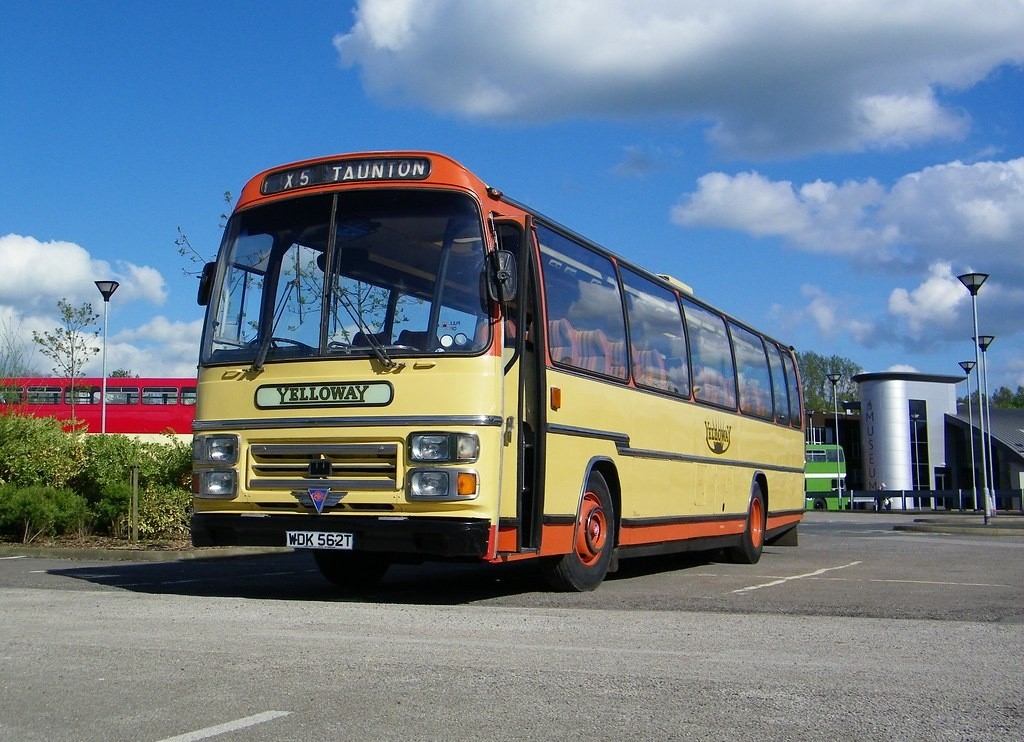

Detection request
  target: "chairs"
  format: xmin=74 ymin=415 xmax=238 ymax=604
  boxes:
xmin=395 ymin=330 xmax=439 ymax=352
xmin=475 ymin=317 xmax=774 ymax=421
xmin=350 ymin=332 xmax=390 ymax=356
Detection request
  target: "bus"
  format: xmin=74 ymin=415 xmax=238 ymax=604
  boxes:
xmin=1 ymin=377 xmax=197 ymax=446
xmin=804 ymin=443 xmax=848 ymax=511
xmin=188 ymin=150 xmax=806 ymax=591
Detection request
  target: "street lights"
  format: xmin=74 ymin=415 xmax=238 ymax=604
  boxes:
xmin=956 ymin=272 xmax=992 ymax=525
xmin=957 ymin=361 xmax=977 ymax=512
xmin=94 ymin=280 xmax=120 ymax=435
xmin=827 ymin=374 xmax=844 ymax=511
xmin=971 ymin=335 xmax=996 ymax=516
